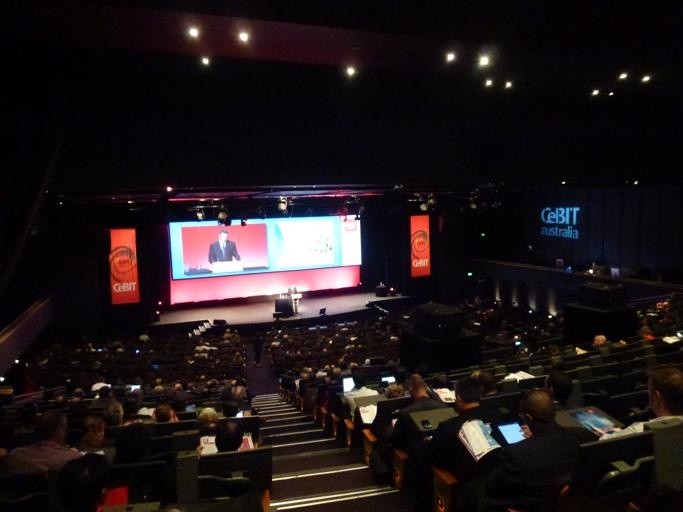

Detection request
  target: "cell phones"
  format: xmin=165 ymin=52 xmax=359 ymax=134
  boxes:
xmin=421 ymin=419 xmax=432 ymax=429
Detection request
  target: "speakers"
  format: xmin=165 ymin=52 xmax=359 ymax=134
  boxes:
xmin=213 ymin=319 xmax=226 ymax=324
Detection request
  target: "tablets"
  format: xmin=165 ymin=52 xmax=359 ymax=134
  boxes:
xmin=494 ymin=421 xmax=526 ymax=445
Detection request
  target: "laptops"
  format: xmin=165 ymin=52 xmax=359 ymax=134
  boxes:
xmin=342 ymin=376 xmax=355 ymax=396
xmin=131 ymin=384 xmax=141 ymax=392
xmin=235 ymin=411 xmax=244 ymax=417
xmin=381 ymin=376 xmax=395 ymax=384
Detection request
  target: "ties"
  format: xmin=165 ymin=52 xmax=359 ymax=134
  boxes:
xmin=223 ymin=244 xmax=225 ymax=256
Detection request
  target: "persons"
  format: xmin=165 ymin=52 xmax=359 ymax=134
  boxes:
xmin=2 ymin=321 xmax=269 ymax=512
xmin=270 ymin=294 xmax=682 ymax=512
xmin=208 ymin=230 xmax=241 ymax=264
